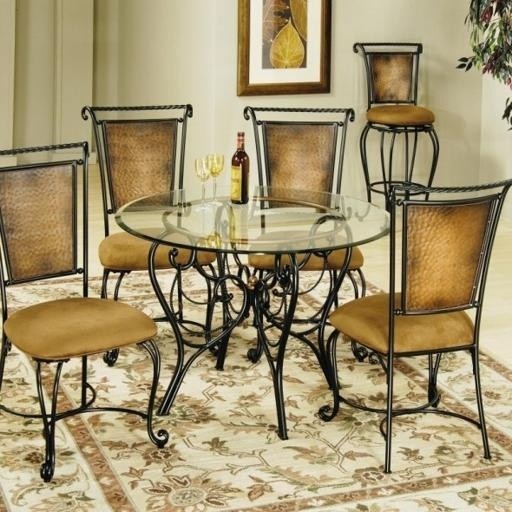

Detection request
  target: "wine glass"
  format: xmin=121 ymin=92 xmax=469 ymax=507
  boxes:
xmin=195 ymin=159 xmax=211 ymax=212
xmin=206 ymin=154 xmax=225 ymax=208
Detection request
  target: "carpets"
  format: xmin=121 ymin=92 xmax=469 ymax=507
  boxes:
xmin=1 ymin=261 xmax=510 ymax=512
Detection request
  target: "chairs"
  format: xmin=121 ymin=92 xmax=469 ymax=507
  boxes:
xmin=80 ymin=103 xmax=219 ymax=366
xmin=2 ymin=141 xmax=169 ymax=484
xmin=240 ymin=104 xmax=369 ymax=362
xmin=352 ymin=41 xmax=439 ymax=216
xmin=315 ymin=176 xmax=511 ymax=475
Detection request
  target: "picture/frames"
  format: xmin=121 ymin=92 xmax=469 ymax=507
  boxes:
xmin=236 ymin=0 xmax=333 ymax=100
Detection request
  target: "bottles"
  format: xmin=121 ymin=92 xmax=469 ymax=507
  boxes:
xmin=228 ymin=204 xmax=248 ymax=251
xmin=230 ymin=132 xmax=250 ymax=204
